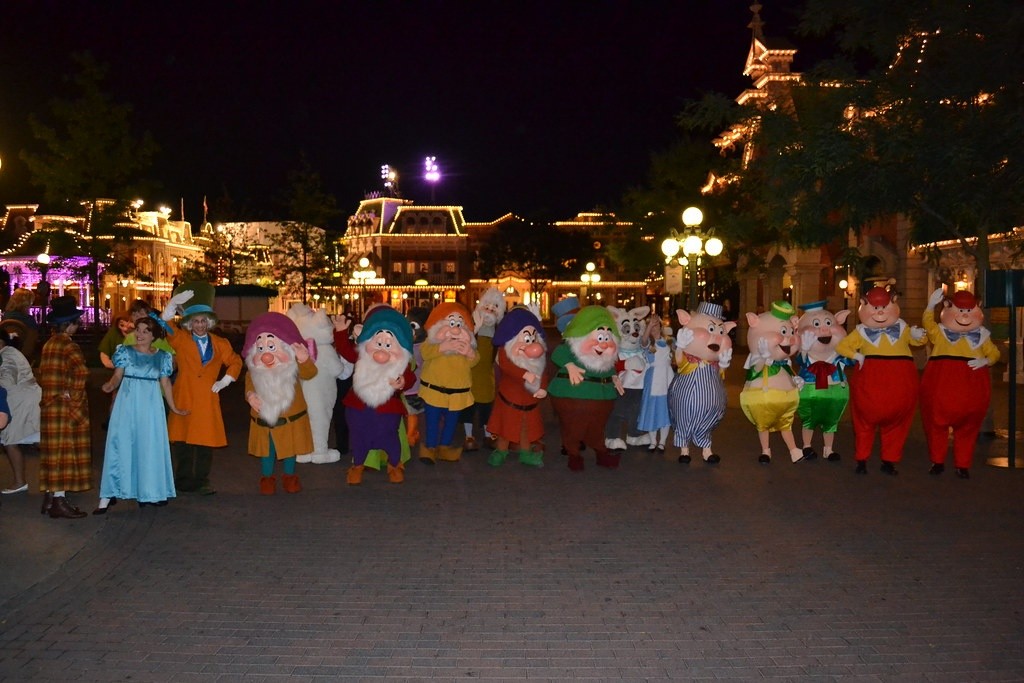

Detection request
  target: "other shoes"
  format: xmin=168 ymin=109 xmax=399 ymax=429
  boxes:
xmin=2 ymin=483 xmax=29 ymax=494
xmin=197 ymin=485 xmax=215 ymax=495
xmin=658 ymin=443 xmax=665 ymax=451
xmin=649 ymin=442 xmax=657 ymax=450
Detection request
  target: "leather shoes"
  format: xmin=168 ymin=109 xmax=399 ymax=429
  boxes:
xmin=41 ymin=492 xmax=55 ymax=514
xmin=51 ymin=496 xmax=88 ymax=518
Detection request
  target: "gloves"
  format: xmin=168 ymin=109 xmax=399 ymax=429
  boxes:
xmin=211 ymin=374 xmax=233 ymax=393
xmin=162 ymin=289 xmax=195 ymax=322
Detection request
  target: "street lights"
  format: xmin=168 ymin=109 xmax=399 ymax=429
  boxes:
xmin=579 ymin=262 xmax=602 ymax=308
xmin=350 ymin=258 xmax=376 ymax=326
xmin=38 ymin=251 xmax=50 ymax=337
xmin=661 ymin=206 xmax=723 ymax=313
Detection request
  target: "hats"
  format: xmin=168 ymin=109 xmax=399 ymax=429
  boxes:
xmin=174 ymin=281 xmax=219 ymax=328
xmin=44 ymin=296 xmax=85 ymax=324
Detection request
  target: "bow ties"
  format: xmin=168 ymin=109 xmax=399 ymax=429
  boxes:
xmin=193 ymin=334 xmax=206 ymax=344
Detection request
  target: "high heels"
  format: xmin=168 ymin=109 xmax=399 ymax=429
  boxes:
xmin=92 ymin=496 xmax=117 ymax=515
xmin=138 ymin=500 xmax=168 ymax=509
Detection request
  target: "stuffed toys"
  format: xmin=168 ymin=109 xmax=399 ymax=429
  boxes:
xmin=738 ymin=299 xmax=808 ymax=465
xmin=793 ymin=298 xmax=850 ymax=462
xmin=404 ymin=306 xmax=428 ymax=448
xmin=665 ymin=302 xmax=736 ymax=463
xmin=918 ymin=286 xmax=1000 ymax=480
xmin=835 ymin=277 xmax=930 ymax=476
xmin=287 ymin=301 xmax=355 ymax=464
xmin=604 ymin=304 xmax=658 ymax=453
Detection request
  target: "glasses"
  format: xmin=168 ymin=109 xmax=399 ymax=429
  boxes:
xmin=192 ymin=318 xmax=208 ymax=325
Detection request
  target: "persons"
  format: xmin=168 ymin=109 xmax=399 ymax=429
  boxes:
xmin=36 ymin=294 xmax=93 ymax=518
xmin=462 ymin=287 xmax=506 ymax=450
xmin=330 ymin=302 xmax=417 ymax=484
xmin=37 ymin=312 xmax=40 ymax=323
xmin=418 ymin=301 xmax=480 ymax=464
xmin=241 ymin=311 xmax=317 ymax=495
xmin=97 ymin=300 xmax=163 ymax=411
xmin=91 ymin=320 xmax=192 ymax=515
xmin=487 ymin=307 xmax=555 ymax=468
xmin=0 ymin=288 xmax=42 ymax=496
xmin=639 ymin=315 xmax=676 ymax=456
xmin=159 ymin=290 xmax=243 ymax=492
xmin=547 ymin=295 xmax=626 ymax=474
